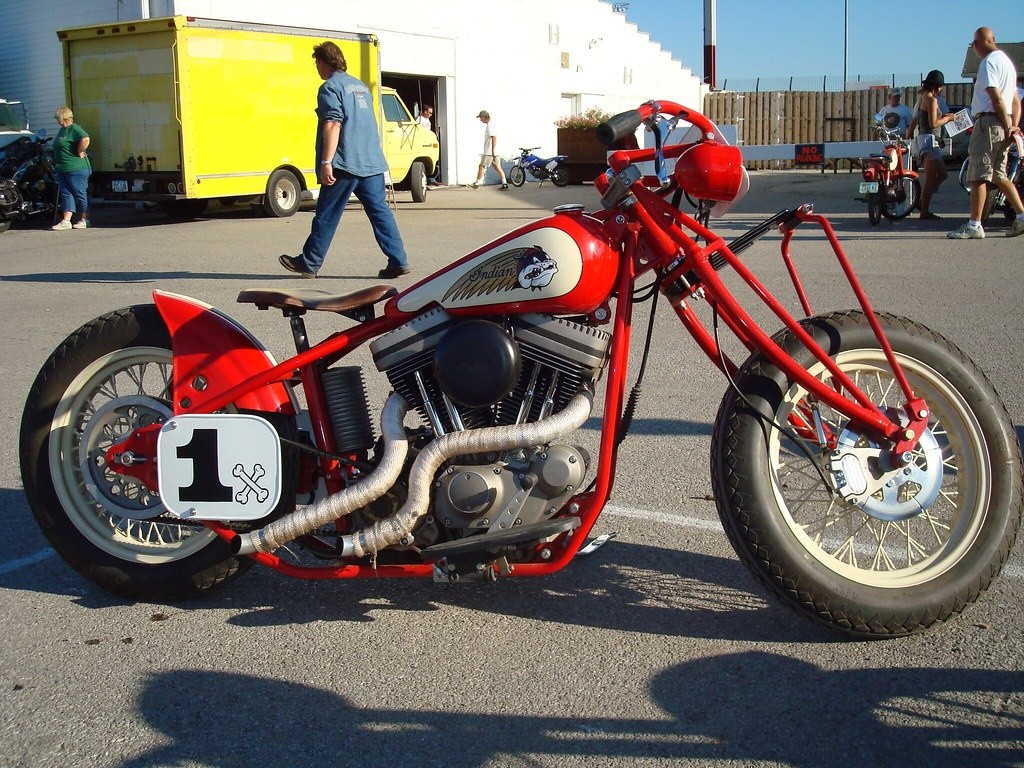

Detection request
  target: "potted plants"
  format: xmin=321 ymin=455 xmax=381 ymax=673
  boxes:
xmin=558 ymin=128 xmax=638 ymax=180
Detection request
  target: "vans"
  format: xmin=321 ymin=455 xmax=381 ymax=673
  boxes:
xmin=0 ymin=98 xmax=38 ymax=158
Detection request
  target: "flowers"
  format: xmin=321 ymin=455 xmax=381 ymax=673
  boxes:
xmin=554 ymin=105 xmax=613 ymax=129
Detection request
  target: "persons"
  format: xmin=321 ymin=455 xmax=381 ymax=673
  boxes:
xmin=909 ymin=69 xmax=958 ymax=220
xmin=415 ymin=105 xmax=439 ymax=186
xmin=51 ymin=107 xmax=92 ymax=230
xmin=466 ymin=110 xmax=508 ymax=191
xmin=278 ymin=42 xmax=409 ymax=278
xmin=874 ymin=87 xmax=911 ymax=139
xmin=949 ymin=27 xmax=1024 ymax=240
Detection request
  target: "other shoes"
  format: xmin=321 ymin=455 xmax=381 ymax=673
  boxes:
xmin=498 ymin=184 xmax=508 ymax=190
xmin=920 ymin=212 xmax=940 ymax=219
xmin=912 ymin=197 xmax=922 ymax=211
xmin=466 ymin=182 xmax=479 ymax=190
xmin=431 ymin=180 xmax=442 ymax=186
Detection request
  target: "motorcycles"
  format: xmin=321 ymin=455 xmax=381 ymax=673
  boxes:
xmin=15 ymin=99 xmax=1024 ymax=642
xmin=509 ymin=146 xmax=572 ymax=189
xmin=959 ymin=129 xmax=1024 ymax=228
xmin=852 ymin=113 xmax=923 ymax=228
xmin=0 ymin=127 xmax=63 ymax=232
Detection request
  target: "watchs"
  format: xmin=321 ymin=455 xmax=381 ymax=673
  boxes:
xmin=321 ymin=160 xmax=331 ymax=165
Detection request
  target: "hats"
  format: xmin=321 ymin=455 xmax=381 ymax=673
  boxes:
xmin=926 ymin=70 xmax=944 ymax=84
xmin=476 ymin=110 xmax=490 ymax=117
xmin=887 ymin=87 xmax=902 ymax=95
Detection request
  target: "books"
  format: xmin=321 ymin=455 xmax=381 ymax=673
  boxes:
xmin=1012 ymin=134 xmax=1024 ymax=157
xmin=944 ymin=109 xmax=973 ymax=137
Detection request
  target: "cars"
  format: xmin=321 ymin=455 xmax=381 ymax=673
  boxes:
xmin=939 ymin=106 xmax=975 ymax=170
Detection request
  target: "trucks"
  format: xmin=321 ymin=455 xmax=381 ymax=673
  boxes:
xmin=55 ymin=13 xmax=441 ymax=218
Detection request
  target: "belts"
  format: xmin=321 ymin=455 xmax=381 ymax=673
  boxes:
xmin=975 ymin=112 xmax=997 ymax=120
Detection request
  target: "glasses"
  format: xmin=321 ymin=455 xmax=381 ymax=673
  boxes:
xmin=426 ymin=111 xmax=433 ymax=114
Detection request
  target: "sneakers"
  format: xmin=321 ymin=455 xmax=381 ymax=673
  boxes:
xmin=278 ymin=254 xmax=315 ymax=278
xmin=73 ymin=220 xmax=86 ymax=229
xmin=1006 ymin=219 xmax=1024 ymax=236
xmin=378 ymin=265 xmax=410 ymax=277
xmin=947 ymin=223 xmax=985 ymax=239
xmin=52 ymin=220 xmax=72 ymax=230
